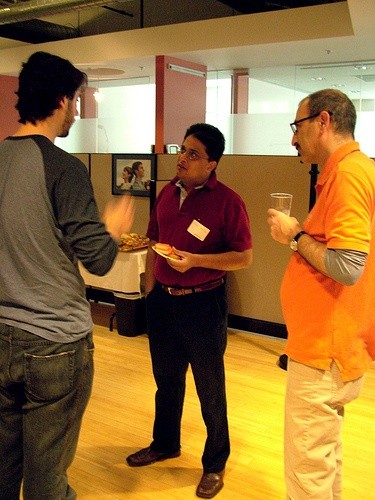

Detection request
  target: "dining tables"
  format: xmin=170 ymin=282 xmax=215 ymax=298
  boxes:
xmin=77 ymin=249 xmax=148 ymax=336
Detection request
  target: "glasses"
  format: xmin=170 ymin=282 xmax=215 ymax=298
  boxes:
xmin=290 ymin=111 xmax=333 ymax=135
xmin=177 ymin=146 xmax=208 ymax=161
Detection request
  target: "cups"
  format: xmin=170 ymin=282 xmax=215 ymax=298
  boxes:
xmin=270 ymin=193 xmax=293 ymax=216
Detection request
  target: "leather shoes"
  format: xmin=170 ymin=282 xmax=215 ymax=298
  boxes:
xmin=196 ymin=465 xmax=225 ymax=499
xmin=126 ymin=445 xmax=180 ymax=467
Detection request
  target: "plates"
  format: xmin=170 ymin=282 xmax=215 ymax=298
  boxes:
xmin=117 ymin=245 xmax=149 ymax=251
xmin=152 ymin=245 xmax=180 ymax=261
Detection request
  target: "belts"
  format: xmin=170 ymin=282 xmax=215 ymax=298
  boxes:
xmin=159 ymin=278 xmax=224 ymax=296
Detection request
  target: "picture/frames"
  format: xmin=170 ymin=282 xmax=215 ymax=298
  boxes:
xmin=111 ymin=153 xmax=157 ymax=196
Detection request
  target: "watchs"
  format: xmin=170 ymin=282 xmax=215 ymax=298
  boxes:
xmin=288 ymin=230 xmax=306 ymax=252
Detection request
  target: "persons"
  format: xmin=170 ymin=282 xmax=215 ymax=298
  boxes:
xmin=0 ymin=50 xmax=116 ymax=499
xmin=266 ymin=88 xmax=373 ymax=500
xmin=125 ymin=124 xmax=253 ymax=498
xmin=118 ymin=167 xmax=134 ymax=190
xmin=130 ymin=161 xmax=146 ymax=190
